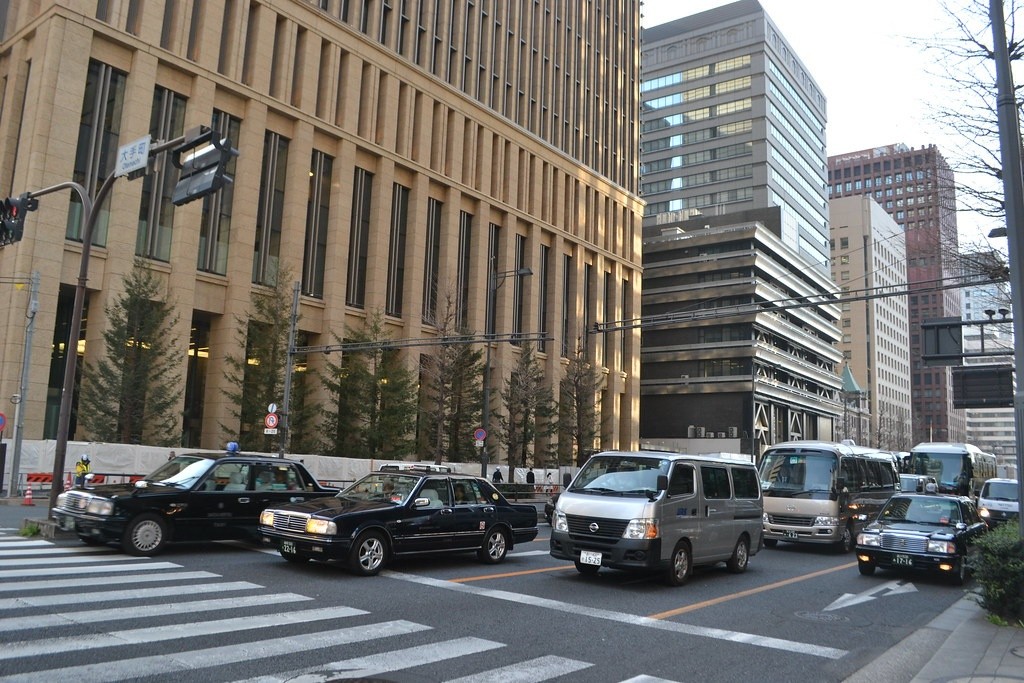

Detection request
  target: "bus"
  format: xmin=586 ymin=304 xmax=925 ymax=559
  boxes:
xmin=755 ymin=439 xmax=998 ymax=552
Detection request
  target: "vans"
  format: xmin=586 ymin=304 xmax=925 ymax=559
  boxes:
xmin=974 ymin=477 xmax=1019 ymax=531
xmin=549 ymin=450 xmax=764 ymax=587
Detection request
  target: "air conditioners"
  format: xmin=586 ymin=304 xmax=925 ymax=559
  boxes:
xmin=728 ymin=426 xmax=737 ymax=437
xmin=706 ymin=432 xmax=714 ymax=438
xmin=681 ymin=375 xmax=689 ymax=378
xmin=718 ymin=432 xmax=726 ymax=438
xmin=699 ymin=253 xmax=707 ymax=257
xmin=696 ymin=427 xmax=706 ymax=438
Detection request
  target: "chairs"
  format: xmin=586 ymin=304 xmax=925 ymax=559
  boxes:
xmin=205 ymin=470 xmax=286 ymax=491
xmin=467 ymin=492 xmax=477 ymax=503
xmin=420 ymin=489 xmax=444 ymax=507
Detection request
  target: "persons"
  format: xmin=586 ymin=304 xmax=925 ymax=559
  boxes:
xmin=455 ymin=484 xmax=467 ymax=505
xmin=493 ymin=467 xmax=503 ymax=483
xmin=76 ymin=455 xmax=90 ymax=485
xmin=256 ymin=469 xmax=296 ymax=490
xmin=378 ymin=480 xmax=396 ymax=498
xmin=526 ymin=467 xmax=535 ymax=483
xmin=168 ymin=451 xmax=180 ymax=476
xmin=546 ymin=473 xmax=553 ymax=496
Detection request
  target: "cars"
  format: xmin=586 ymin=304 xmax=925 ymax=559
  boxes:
xmin=855 ymin=491 xmax=990 ymax=587
xmin=256 ymin=463 xmax=539 ymax=577
xmin=51 ymin=441 xmax=345 ymax=557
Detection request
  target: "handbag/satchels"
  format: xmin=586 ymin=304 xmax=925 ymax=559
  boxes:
xmin=549 ymin=475 xmax=554 ymax=484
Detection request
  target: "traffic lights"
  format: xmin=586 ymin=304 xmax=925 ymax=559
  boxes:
xmin=170 ymin=138 xmax=240 ymax=206
xmin=0 ymin=197 xmax=26 ymax=247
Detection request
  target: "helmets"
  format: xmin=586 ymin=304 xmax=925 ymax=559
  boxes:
xmin=80 ymin=453 xmax=92 ymax=462
xmin=496 ymin=466 xmax=501 ymax=471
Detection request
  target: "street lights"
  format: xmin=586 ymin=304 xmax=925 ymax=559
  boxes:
xmin=844 ymin=395 xmax=867 ymax=438
xmin=481 ymin=267 xmax=533 ymax=478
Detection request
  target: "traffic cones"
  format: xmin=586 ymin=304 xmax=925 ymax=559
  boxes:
xmin=21 ymin=480 xmax=35 ymax=505
xmin=64 ymin=472 xmax=71 ymax=491
